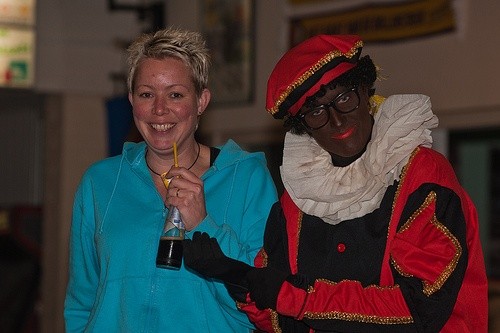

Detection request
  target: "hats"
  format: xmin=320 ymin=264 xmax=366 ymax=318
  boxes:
xmin=264 ymin=34 xmax=366 ymax=121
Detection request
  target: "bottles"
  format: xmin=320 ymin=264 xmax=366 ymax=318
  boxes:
xmin=155 ymin=205 xmax=186 ymax=271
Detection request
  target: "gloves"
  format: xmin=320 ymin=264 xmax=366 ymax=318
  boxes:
xmin=242 ymin=266 xmax=284 ymax=310
xmin=181 ymin=231 xmax=234 ymax=285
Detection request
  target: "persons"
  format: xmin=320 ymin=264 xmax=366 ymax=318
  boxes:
xmin=183 ymin=35 xmax=488 ymax=333
xmin=64 ymin=27 xmax=277 ymax=333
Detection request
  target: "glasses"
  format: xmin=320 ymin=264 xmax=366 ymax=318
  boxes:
xmin=296 ymin=81 xmax=363 ymax=131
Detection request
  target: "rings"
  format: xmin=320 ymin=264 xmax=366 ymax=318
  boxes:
xmin=175 ymin=188 xmax=181 ymax=198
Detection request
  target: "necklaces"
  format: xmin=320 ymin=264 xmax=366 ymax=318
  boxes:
xmin=144 ymin=141 xmax=201 ymax=177
